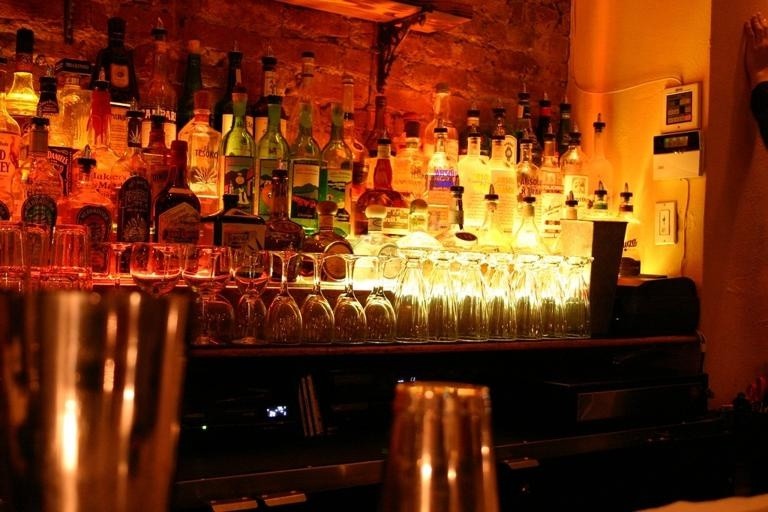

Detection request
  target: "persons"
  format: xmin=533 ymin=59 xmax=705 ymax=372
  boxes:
xmin=743 ymin=11 xmax=768 ymax=146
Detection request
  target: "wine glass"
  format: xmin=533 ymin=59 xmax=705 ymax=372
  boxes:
xmin=1 ymin=218 xmax=588 ymax=347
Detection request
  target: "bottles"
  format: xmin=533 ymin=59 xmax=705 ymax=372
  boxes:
xmin=1 ymin=16 xmax=643 ymax=288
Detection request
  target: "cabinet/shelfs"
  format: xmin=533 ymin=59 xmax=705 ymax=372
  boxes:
xmin=167 ymin=283 xmax=716 ymax=512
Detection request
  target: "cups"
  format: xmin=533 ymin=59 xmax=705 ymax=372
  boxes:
xmin=2 ymin=288 xmax=186 ymax=512
xmin=380 ymin=378 xmax=501 ymax=512
